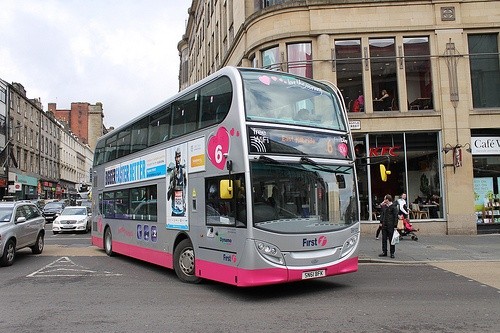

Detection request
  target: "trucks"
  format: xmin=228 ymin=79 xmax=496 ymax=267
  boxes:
xmin=74 ymin=183 xmax=92 ymax=207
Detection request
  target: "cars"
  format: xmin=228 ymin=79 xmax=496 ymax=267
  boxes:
xmin=51 ymin=205 xmax=92 ymax=235
xmin=30 ymin=198 xmax=71 ymax=212
xmin=41 ymin=201 xmax=65 ymax=224
xmin=81 ymin=199 xmax=92 ymax=213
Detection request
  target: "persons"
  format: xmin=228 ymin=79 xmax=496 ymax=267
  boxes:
xmin=414 ymin=194 xmax=439 ymax=218
xmin=297 ymin=109 xmax=310 ymax=120
xmin=368 ymin=195 xmax=380 ymax=220
xmin=376 ymin=201 xmax=388 ymax=240
xmin=378 ymin=90 xmax=392 ymax=112
xmin=378 ymin=195 xmax=398 ymax=258
xmin=396 ymin=193 xmax=409 ymax=240
xmin=270 ymin=187 xmax=281 ymax=207
xmin=167 ymin=147 xmax=186 ymax=216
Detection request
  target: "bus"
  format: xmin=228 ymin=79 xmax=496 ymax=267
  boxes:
xmin=89 ymin=64 xmax=393 ymax=291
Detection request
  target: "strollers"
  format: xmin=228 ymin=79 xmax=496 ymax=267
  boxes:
xmin=396 ymin=213 xmax=420 ymax=241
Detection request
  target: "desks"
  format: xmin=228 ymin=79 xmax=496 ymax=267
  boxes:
xmin=373 ymin=99 xmax=383 ymax=111
xmin=410 ymin=98 xmax=431 ymax=109
xmin=419 ymin=204 xmax=437 ymax=219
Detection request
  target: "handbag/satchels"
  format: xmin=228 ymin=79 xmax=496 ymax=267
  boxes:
xmin=391 ymin=229 xmax=400 ymax=245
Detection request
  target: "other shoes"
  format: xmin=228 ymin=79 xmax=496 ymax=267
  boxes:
xmin=391 ymin=253 xmax=394 ymax=258
xmin=376 ymin=237 xmax=378 ymax=240
xmin=379 ymin=253 xmax=387 ymax=256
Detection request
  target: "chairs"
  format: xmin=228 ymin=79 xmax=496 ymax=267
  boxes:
xmin=108 ymin=102 xmax=230 ymax=162
xmin=411 ymin=204 xmax=428 ymax=219
xmin=382 ymin=97 xmax=394 ymax=111
xmin=366 ymin=205 xmax=378 ymax=221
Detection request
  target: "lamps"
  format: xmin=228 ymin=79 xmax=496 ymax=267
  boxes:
xmin=444 ymin=143 xmax=453 ymax=154
xmin=455 ymin=143 xmax=472 ymax=154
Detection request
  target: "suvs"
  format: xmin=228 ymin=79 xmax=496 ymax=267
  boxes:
xmin=0 ymin=194 xmax=46 ymax=267
